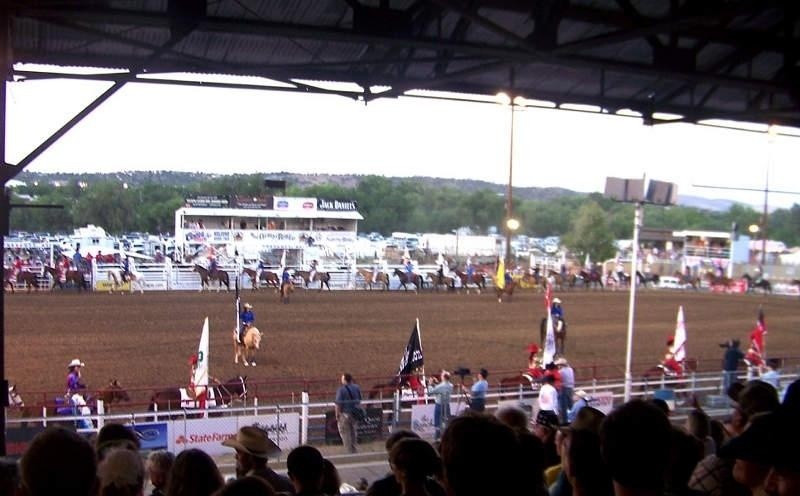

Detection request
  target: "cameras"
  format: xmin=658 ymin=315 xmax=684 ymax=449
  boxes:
xmin=719 ymin=338 xmax=730 ymax=350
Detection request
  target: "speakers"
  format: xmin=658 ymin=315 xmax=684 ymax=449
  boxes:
xmin=671 ymin=184 xmax=680 ymax=204
xmin=604 ymin=176 xmax=627 ymax=200
xmin=626 ymin=179 xmax=646 ymax=202
xmin=647 ymin=179 xmax=670 ymax=206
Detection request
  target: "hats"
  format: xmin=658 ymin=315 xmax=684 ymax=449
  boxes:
xmin=68 ymin=359 xmax=85 ymax=367
xmin=542 ymin=357 xmax=568 ymax=382
xmin=575 ymin=390 xmax=587 ymax=397
xmin=95 ymin=423 xmax=145 ymax=493
xmin=244 ymin=303 xmax=253 ymax=309
xmin=222 ymin=426 xmax=281 ymax=457
xmin=728 ymin=379 xmax=779 ymax=412
xmin=717 ymin=381 xmax=798 ymax=474
xmin=552 ymin=297 xmax=562 ymax=303
xmin=527 ymin=342 xmax=542 ymax=353
xmin=551 ymin=407 xmax=604 ymax=441
xmin=536 ymin=409 xmax=557 ymax=428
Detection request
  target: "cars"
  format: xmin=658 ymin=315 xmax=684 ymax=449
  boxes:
xmin=363 ymin=228 xmax=595 ymax=268
xmin=652 ymin=274 xmax=693 ymax=288
xmin=4 ymin=226 xmax=176 ymax=265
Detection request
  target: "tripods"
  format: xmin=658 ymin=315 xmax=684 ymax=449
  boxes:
xmin=449 ymin=379 xmax=473 ymax=422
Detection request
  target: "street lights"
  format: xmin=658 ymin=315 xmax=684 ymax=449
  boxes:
xmin=603 ymin=176 xmax=678 ymax=406
xmin=749 ymin=222 xmax=760 ymax=268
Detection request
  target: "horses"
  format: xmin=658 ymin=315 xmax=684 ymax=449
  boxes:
xmin=241 ymin=266 xmax=332 ymax=304
xmin=192 ymin=262 xmax=231 ymax=295
xmin=8 ymin=383 xmax=25 ymax=410
xmin=356 ymin=264 xmax=485 ymax=294
xmin=232 ymin=327 xmax=264 ymax=367
xmin=22 ymin=380 xmax=130 ymax=428
xmin=145 ymin=375 xmax=248 ymax=422
xmin=366 ymin=383 xmax=417 ymax=426
xmin=5 ymin=266 xmax=147 ymax=295
xmin=521 ymin=270 xmax=661 ymax=292
xmin=637 ymin=358 xmax=698 ymax=398
xmin=499 ymin=374 xmax=539 ymax=400
xmin=541 ymin=317 xmax=565 ymax=355
xmin=673 ymin=268 xmax=772 ymax=294
xmin=495 ymin=280 xmax=517 ymax=303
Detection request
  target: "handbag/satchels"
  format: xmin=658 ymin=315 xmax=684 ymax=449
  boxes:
xmin=351 ymin=405 xmax=365 ymax=421
